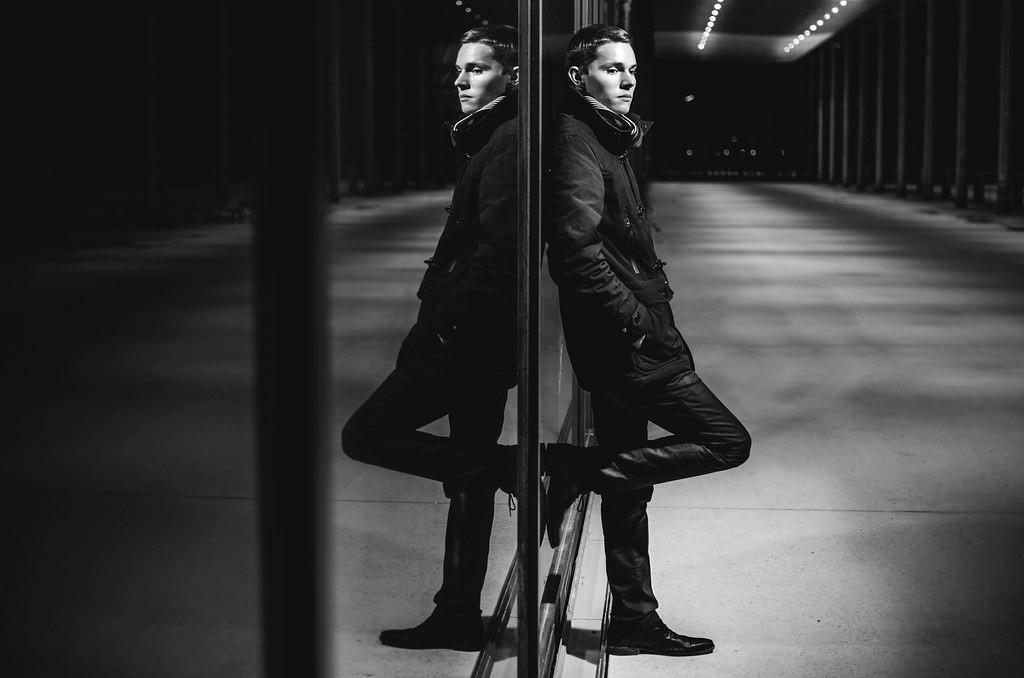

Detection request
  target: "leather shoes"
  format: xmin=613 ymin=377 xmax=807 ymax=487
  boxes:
xmin=509 ymin=444 xmax=546 ymax=546
xmin=608 ymin=618 xmax=715 ymax=655
xmin=547 ymin=443 xmax=585 ymax=549
xmin=380 ymin=608 xmax=485 ymax=653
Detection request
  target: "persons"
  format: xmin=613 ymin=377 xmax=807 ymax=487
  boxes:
xmin=342 ymin=24 xmax=546 ymax=652
xmin=545 ymin=23 xmax=753 ymax=655
